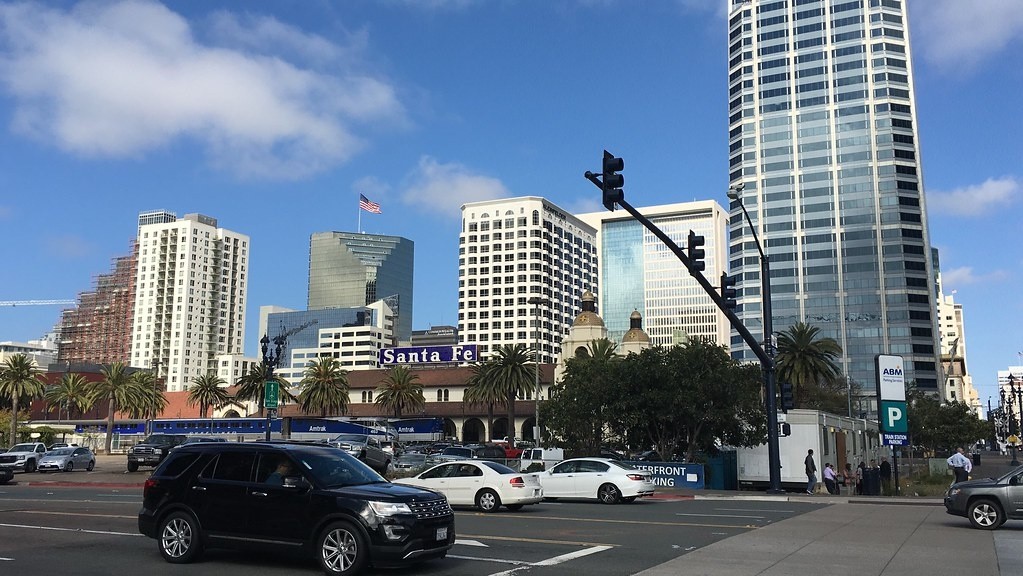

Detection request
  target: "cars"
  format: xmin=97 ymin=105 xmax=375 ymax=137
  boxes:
xmin=36 ymin=447 xmax=96 ymax=473
xmin=391 ymin=460 xmax=544 ymax=513
xmin=386 ymin=436 xmax=564 ymax=478
xmin=600 ymin=450 xmax=660 ymax=460
xmin=527 ymin=457 xmax=656 ymax=505
xmin=0 ymin=465 xmax=14 ymax=484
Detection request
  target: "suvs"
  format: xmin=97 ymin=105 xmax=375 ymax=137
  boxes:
xmin=943 ymin=464 xmax=1023 ymax=531
xmin=173 ymin=436 xmax=227 ymax=449
xmin=47 ymin=443 xmax=78 ymax=451
xmin=126 ymin=434 xmax=188 ymax=472
xmin=137 ymin=442 xmax=455 ymax=576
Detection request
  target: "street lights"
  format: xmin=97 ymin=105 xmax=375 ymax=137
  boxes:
xmin=527 ymin=298 xmax=548 ymax=449
xmin=150 ymin=361 xmax=164 ymax=434
xmin=1000 ymin=372 xmax=1023 ymax=466
xmin=259 ymin=332 xmax=284 ymax=443
xmin=725 ymin=188 xmax=787 ymax=495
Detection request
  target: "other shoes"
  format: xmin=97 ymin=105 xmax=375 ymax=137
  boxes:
xmin=807 ymin=490 xmax=813 ymax=494
xmin=807 ymin=493 xmax=812 ymax=496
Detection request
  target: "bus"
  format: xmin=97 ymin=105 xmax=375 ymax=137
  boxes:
xmin=16 ymin=417 xmax=445 ymax=452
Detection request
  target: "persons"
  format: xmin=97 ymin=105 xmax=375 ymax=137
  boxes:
xmin=947 ymin=447 xmax=972 ymax=484
xmin=880 ymin=456 xmax=891 ymax=496
xmin=265 ymin=456 xmax=292 ymax=485
xmin=843 ymin=463 xmax=856 ymax=497
xmin=805 ymin=449 xmax=817 ymax=495
xmin=854 ymin=461 xmax=866 ymax=494
xmin=823 ymin=462 xmax=840 ymax=495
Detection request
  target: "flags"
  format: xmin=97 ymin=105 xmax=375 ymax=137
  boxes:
xmin=359 ymin=193 xmax=382 ymax=214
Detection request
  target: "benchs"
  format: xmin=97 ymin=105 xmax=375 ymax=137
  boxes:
xmin=835 ymin=476 xmax=857 ymax=495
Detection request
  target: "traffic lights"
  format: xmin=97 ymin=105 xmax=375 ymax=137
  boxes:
xmin=602 ymin=149 xmax=625 ymax=212
xmin=720 ymin=270 xmax=736 ymax=310
xmin=778 ymin=377 xmax=794 ymax=414
xmin=688 ymin=229 xmax=706 ymax=277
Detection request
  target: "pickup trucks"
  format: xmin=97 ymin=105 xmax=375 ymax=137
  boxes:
xmin=0 ymin=442 xmax=51 ymax=473
xmin=326 ymin=433 xmax=393 ymax=475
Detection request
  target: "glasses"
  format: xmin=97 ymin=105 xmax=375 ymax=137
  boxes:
xmin=281 ymin=465 xmax=290 ymax=468
